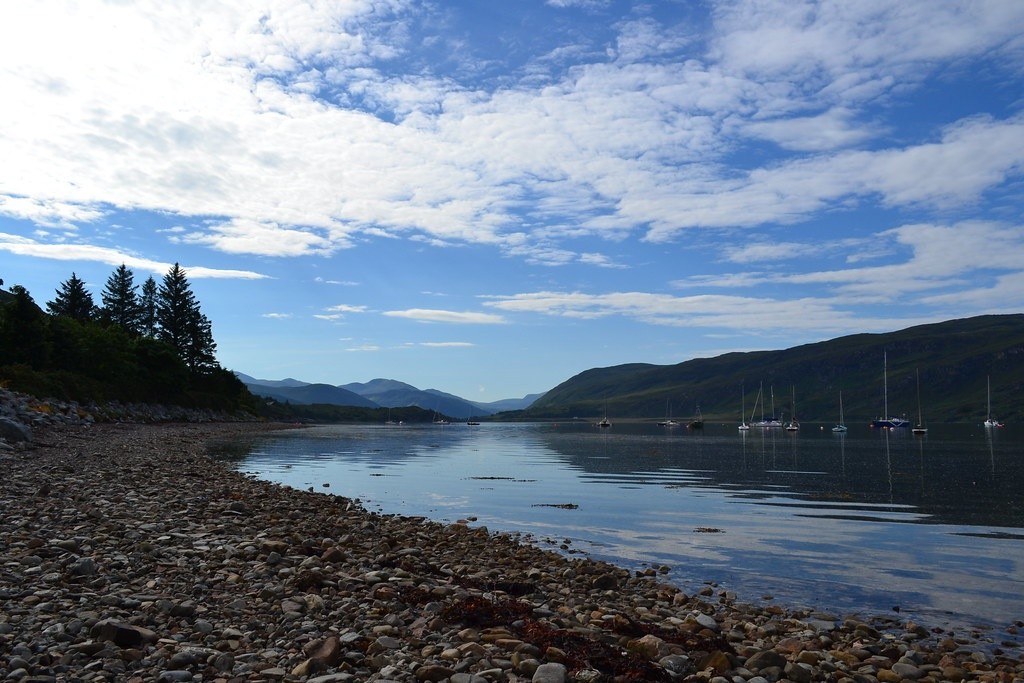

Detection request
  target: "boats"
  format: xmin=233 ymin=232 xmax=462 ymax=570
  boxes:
xmin=466 ymin=407 xmax=480 ymax=425
xmin=872 ymin=352 xmax=910 ymax=428
xmin=983 ymin=375 xmax=999 ymax=428
xmin=749 ymin=380 xmax=783 ymax=426
xmin=912 ymin=368 xmax=928 ymax=434
xmin=656 ymin=396 xmax=681 ymax=427
xmin=686 ymin=406 xmax=704 ymax=428
xmin=832 ymin=390 xmax=847 ymax=432
xmin=785 ymin=385 xmax=800 ymax=431
xmin=593 ymin=399 xmax=611 ymax=428
xmin=738 ymin=385 xmax=750 ymax=430
xmin=432 ymin=400 xmax=450 ymax=425
xmin=385 ymin=406 xmax=406 ymax=426
